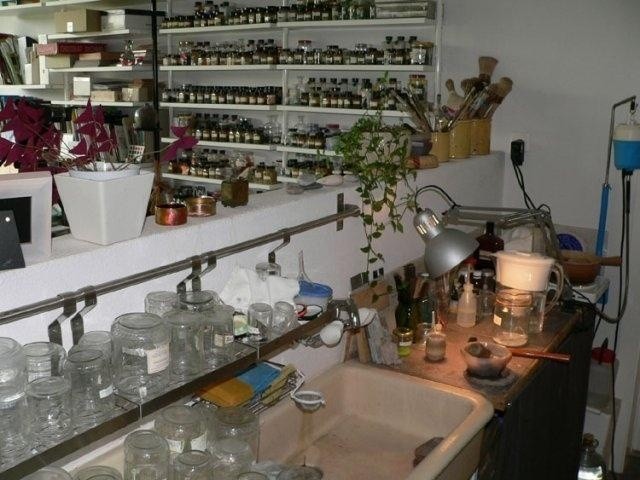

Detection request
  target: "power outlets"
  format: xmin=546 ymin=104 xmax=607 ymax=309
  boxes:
xmin=507 ymin=130 xmax=531 ymax=154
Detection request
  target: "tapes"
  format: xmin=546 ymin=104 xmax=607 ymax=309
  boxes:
xmin=186 ymin=195 xmax=216 ymax=217
xmin=155 ymin=203 xmax=187 ymax=225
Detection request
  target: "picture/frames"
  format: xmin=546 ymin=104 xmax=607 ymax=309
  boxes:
xmin=0 ymin=169 xmax=55 ymax=272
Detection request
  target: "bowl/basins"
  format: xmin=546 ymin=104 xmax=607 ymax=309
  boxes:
xmin=457 ymin=342 xmax=511 ymax=377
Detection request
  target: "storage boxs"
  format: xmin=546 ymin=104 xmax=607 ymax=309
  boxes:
xmin=50 ymin=7 xmax=103 ymax=35
xmin=71 ymin=76 xmax=94 ymax=98
xmin=120 ymin=86 xmax=149 ymax=102
xmin=100 ymin=7 xmax=155 ymax=31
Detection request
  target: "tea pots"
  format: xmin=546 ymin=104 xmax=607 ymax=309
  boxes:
xmin=490 ymin=249 xmax=563 ymax=333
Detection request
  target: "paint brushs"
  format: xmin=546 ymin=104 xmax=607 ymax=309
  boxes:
xmin=390 ymin=86 xmax=478 ymax=133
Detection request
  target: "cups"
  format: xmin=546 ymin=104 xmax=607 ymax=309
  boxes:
xmin=430 ymin=131 xmax=450 ymax=164
xmin=449 ymin=118 xmax=472 ymax=159
xmin=472 ymin=118 xmax=491 ymax=155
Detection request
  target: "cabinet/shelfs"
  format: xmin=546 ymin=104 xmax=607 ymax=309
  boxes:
xmin=150 ymin=1 xmax=446 ymax=195
xmin=0 ymin=295 xmax=337 ymax=480
xmin=1 ymin=1 xmax=70 ymax=91
xmin=44 ymin=0 xmax=153 ymax=108
xmin=389 ymin=298 xmax=592 ymax=479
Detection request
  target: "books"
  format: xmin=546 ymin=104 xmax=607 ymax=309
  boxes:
xmin=0 ymin=32 xmax=168 ymax=164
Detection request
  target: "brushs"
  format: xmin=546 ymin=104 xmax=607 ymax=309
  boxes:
xmin=445 ymin=56 xmax=512 ymax=121
xmin=293 ymin=281 xmax=333 ymax=305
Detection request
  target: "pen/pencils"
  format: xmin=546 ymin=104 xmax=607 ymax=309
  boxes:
xmin=393 ymin=263 xmax=429 ymax=299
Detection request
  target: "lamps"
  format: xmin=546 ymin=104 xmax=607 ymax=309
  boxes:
xmin=407 ymin=183 xmax=569 ymax=284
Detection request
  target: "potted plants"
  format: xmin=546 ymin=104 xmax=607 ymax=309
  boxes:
xmin=324 ymin=66 xmax=422 ymax=304
xmin=0 ymin=96 xmax=201 ymax=181
xmin=209 ymin=148 xmax=251 ymax=207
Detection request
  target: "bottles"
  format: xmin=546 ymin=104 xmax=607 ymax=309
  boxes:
xmin=286 ymin=114 xmax=342 ymax=153
xmin=285 ymin=158 xmax=333 ymax=179
xmin=426 ymin=331 xmax=447 ymax=361
xmin=395 ymin=298 xmax=425 ymax=345
xmin=162 ymin=82 xmax=281 ymax=105
xmin=291 ymin=75 xmax=428 ymax=108
xmin=192 ymin=112 xmax=277 ymax=144
xmin=492 ymin=288 xmax=531 ymax=348
xmin=391 ymin=327 xmax=413 ymax=357
xmin=587 ymin=336 xmax=617 ymax=408
xmin=419 ymin=281 xmax=438 ymax=324
xmin=161 ymin=0 xmax=375 ymax=30
xmin=167 ymin=148 xmax=282 ymax=186
xmin=22 ymin=404 xmax=324 ymax=479
xmin=474 ymin=221 xmax=505 ymax=275
xmin=571 ymin=431 xmax=607 ymax=479
xmin=163 ymin=35 xmax=433 ymax=67
xmin=0 ymin=287 xmax=239 ymax=468
xmin=247 ymin=299 xmax=292 ymax=341
xmin=454 ymin=262 xmax=493 ymax=327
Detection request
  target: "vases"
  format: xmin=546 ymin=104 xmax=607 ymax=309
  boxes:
xmin=54 ymin=170 xmax=157 ymax=247
